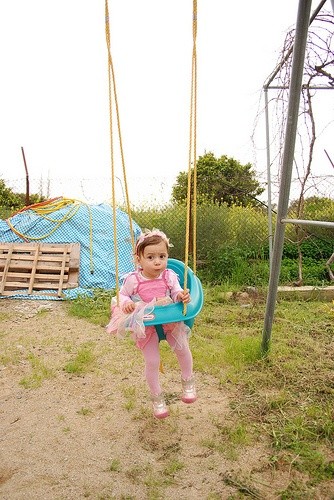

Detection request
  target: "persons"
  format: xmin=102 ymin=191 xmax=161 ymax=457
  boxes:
xmin=104 ymin=228 xmax=203 ymax=417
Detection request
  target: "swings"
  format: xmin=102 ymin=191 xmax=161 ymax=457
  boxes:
xmin=99 ymin=0 xmax=201 ymax=342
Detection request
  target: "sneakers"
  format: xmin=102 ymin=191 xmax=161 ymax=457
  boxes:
xmin=150 ymin=392 xmax=168 ymax=419
xmin=181 ymin=376 xmax=197 ymax=403
